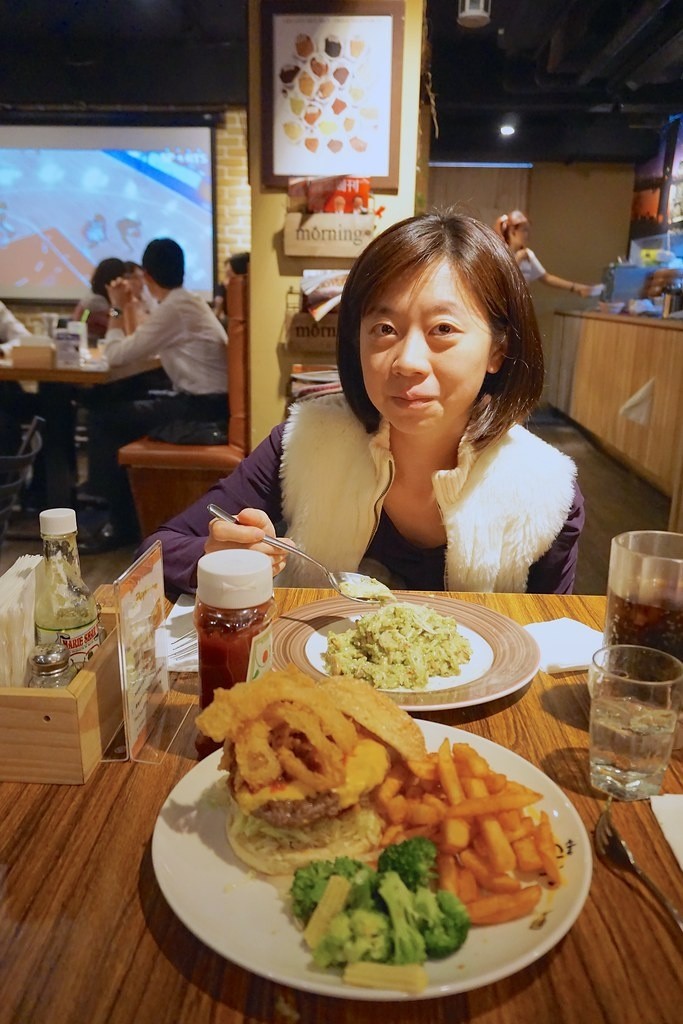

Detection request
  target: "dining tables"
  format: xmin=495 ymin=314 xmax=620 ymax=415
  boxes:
xmin=0 ymin=583 xmax=682 ymax=1023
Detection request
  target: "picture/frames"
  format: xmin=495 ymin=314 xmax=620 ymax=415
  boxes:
xmin=259 ymin=1 xmax=400 ymax=192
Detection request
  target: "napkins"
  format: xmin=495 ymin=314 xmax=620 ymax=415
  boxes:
xmin=520 ymin=616 xmax=607 ymax=675
xmin=153 ymin=592 xmax=202 ymax=673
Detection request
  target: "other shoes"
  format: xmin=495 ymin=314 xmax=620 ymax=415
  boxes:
xmin=75 ymin=481 xmax=110 ymax=508
xmin=99 ymin=518 xmax=121 ymax=548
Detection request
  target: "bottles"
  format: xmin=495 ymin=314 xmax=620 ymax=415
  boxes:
xmin=191 ymin=548 xmax=276 ymax=764
xmin=29 ymin=642 xmax=78 ymax=689
xmin=32 ymin=508 xmax=103 ymax=675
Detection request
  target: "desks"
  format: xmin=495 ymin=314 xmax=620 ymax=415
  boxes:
xmin=1 ymin=346 xmax=164 ymax=544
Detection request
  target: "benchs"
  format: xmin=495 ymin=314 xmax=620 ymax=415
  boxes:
xmin=114 ymin=274 xmax=251 ymax=529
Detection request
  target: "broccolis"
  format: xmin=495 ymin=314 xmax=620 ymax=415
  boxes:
xmin=287 ymin=833 xmax=469 ymax=967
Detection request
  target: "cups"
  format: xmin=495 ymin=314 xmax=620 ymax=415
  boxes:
xmin=66 ymin=321 xmax=89 ymax=361
xmin=589 ymin=643 xmax=683 ymax=802
xmin=604 ymin=529 xmax=683 ymax=752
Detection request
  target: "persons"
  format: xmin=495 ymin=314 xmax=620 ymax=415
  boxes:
xmin=0 ymin=299 xmax=33 ymax=357
xmin=77 ymin=237 xmax=251 ymax=555
xmin=497 ymin=209 xmax=603 ymax=311
xmin=131 ymin=212 xmax=585 ymax=605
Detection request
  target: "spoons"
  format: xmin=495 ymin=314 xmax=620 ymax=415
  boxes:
xmin=206 ymin=503 xmax=393 ymax=603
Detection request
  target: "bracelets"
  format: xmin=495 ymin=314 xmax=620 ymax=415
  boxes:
xmin=109 ymin=307 xmax=122 ymax=318
xmin=570 ymin=282 xmax=576 ymax=292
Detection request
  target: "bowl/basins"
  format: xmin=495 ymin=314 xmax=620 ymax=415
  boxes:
xmin=597 ymin=301 xmax=625 ymax=315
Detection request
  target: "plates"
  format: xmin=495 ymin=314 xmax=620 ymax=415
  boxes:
xmin=151 ymin=719 xmax=594 ymax=1003
xmin=269 ymin=591 xmax=542 ymax=712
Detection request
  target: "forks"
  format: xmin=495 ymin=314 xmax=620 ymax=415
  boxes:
xmin=595 ymin=811 xmax=683 ymax=933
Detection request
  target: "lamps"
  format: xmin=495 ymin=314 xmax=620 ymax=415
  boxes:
xmin=501 ymin=112 xmax=520 ymax=134
xmin=457 ymin=0 xmax=491 ymax=27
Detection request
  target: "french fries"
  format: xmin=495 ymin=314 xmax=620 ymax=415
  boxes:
xmin=378 ymin=738 xmax=561 ymax=923
